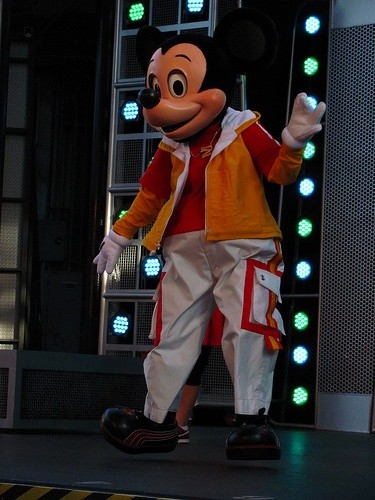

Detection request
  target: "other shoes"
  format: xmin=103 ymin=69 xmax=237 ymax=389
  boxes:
xmin=178 ymin=425 xmax=190 ymax=443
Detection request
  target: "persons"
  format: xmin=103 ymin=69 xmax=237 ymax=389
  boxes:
xmin=175 ymin=304 xmax=225 ymax=444
xmin=92 ymin=25 xmax=328 ymax=461
